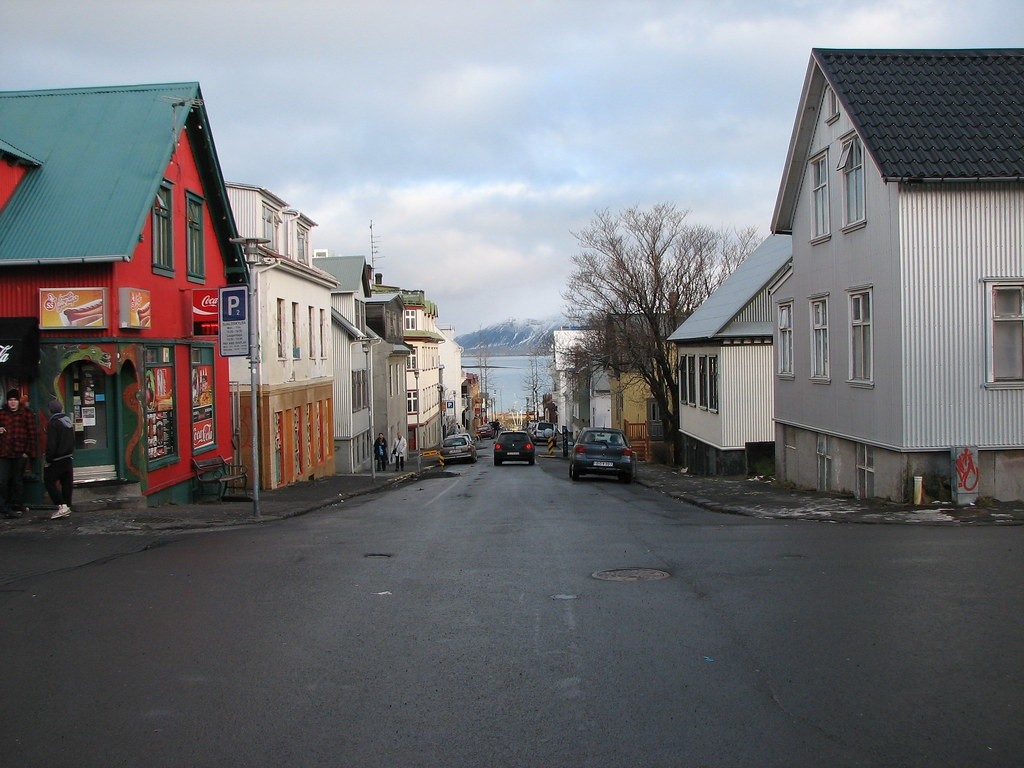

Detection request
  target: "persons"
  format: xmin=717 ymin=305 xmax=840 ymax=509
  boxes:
xmin=374 ymin=433 xmax=388 ymax=471
xmin=491 ymin=419 xmax=500 ymax=438
xmin=44 ymin=400 xmax=74 ymax=519
xmin=392 ymin=432 xmax=407 ymax=472
xmin=0 ymin=389 xmax=36 ymax=517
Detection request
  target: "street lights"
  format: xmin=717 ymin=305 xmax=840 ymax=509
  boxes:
xmin=231 ymin=231 xmax=273 ymax=517
xmin=413 ymin=369 xmax=422 ymax=474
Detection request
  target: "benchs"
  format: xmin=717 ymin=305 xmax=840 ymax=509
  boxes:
xmin=192 ymin=455 xmax=249 ymax=504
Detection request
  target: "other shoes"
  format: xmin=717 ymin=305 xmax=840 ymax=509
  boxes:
xmin=395 ymin=468 xmax=399 ymax=472
xmin=377 ymin=468 xmax=381 ymax=471
xmin=14 ymin=503 xmax=25 ymax=513
xmin=383 ymin=468 xmax=385 ymax=471
xmin=402 ymin=468 xmax=404 ymax=471
xmin=0 ymin=504 xmax=10 ymax=514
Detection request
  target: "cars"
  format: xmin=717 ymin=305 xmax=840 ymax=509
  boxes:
xmin=489 ymin=422 xmax=508 ymax=431
xmin=477 ymin=425 xmax=494 ymax=439
xmin=569 ymin=427 xmax=637 ymax=484
xmin=418 ymin=450 xmax=440 ymax=458
xmin=492 ymin=431 xmax=535 ymax=465
xmin=440 ymin=433 xmax=478 ymax=463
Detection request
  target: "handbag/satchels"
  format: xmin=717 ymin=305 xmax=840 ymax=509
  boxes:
xmin=393 ymin=448 xmax=396 ymax=454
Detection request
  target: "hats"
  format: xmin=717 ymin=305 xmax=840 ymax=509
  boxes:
xmin=48 ymin=400 xmax=61 ymax=413
xmin=7 ymin=389 xmax=19 ymax=400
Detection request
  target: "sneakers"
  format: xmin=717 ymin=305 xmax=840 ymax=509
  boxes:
xmin=50 ymin=504 xmax=71 ymax=520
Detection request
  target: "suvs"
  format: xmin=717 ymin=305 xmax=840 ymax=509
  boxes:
xmin=529 ymin=422 xmax=558 ymax=445
